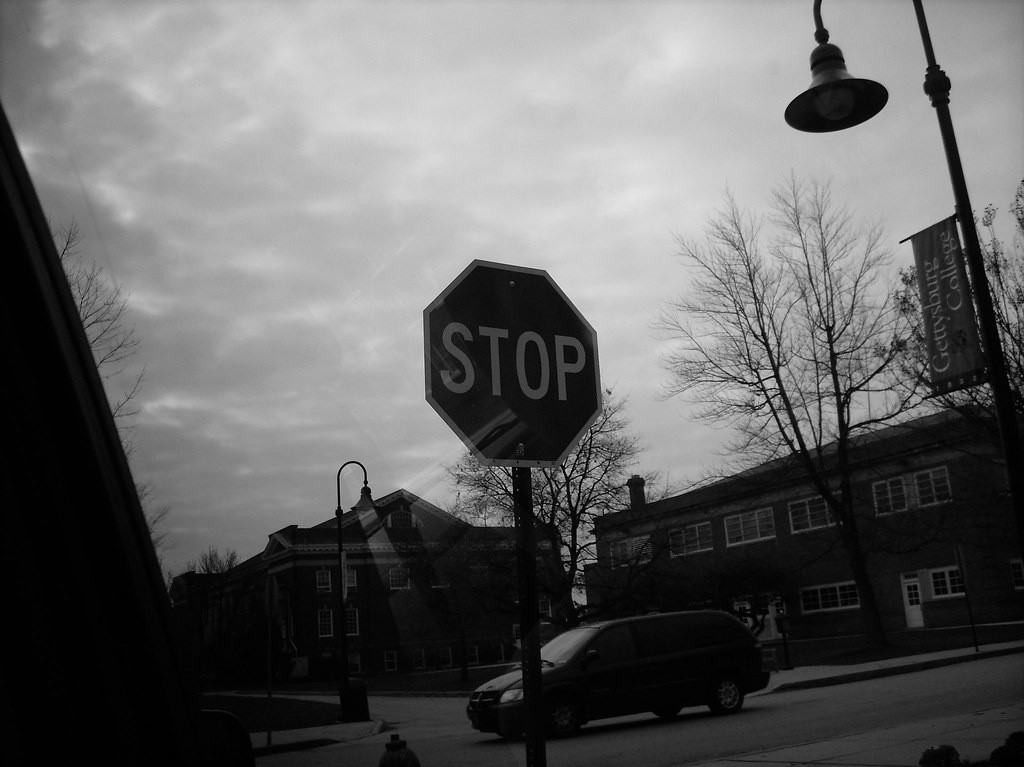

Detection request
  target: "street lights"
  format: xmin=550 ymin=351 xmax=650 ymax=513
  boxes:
xmin=784 ymin=1 xmax=1023 ymax=545
xmin=335 ymin=460 xmax=381 ymax=722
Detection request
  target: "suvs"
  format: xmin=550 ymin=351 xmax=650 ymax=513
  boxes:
xmin=466 ymin=610 xmax=771 ymax=740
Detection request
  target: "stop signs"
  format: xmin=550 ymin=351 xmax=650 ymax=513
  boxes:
xmin=425 ymin=257 xmax=603 ymax=469
